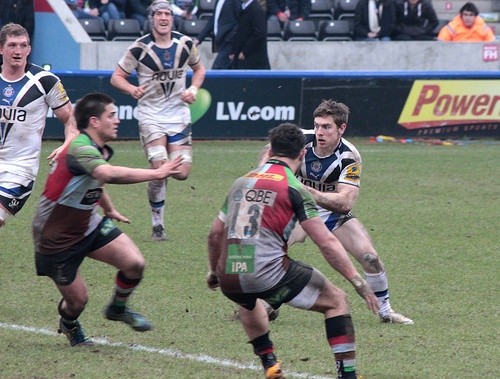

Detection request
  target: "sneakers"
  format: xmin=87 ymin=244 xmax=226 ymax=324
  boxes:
xmin=102 ymin=301 xmax=151 ymax=332
xmin=380 ymin=310 xmax=414 ymax=324
xmin=152 ymin=229 xmax=166 ymax=240
xmin=59 ymin=317 xmax=85 ymax=347
xmin=264 ymin=353 xmax=283 ymax=378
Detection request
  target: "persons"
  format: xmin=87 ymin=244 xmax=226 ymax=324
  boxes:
xmin=256 ymin=98 xmax=415 ymax=325
xmin=205 ymin=124 xmax=380 ymax=379
xmin=111 ymin=0 xmax=206 ymax=241
xmin=77 ymin=0 xmax=439 ymax=70
xmin=0 ymin=23 xmax=79 ymax=227
xmin=31 ymin=92 xmax=186 ymax=346
xmin=437 ymin=2 xmax=496 ymax=42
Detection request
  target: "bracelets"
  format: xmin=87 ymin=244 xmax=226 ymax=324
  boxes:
xmin=188 ymin=85 xmax=199 ymax=95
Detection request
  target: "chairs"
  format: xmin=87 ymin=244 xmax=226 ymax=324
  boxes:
xmin=65 ymin=0 xmax=450 ymax=42
